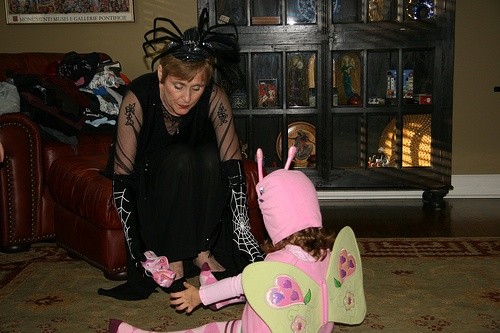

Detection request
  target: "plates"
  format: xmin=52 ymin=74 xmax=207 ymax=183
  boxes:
xmin=276 ymin=122 xmax=317 ymax=168
xmin=297 ymin=0 xmax=337 ymax=22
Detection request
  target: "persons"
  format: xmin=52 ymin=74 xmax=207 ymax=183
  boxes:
xmin=109 ymin=145 xmax=335 ymax=333
xmin=97 ymin=8 xmax=267 ymax=300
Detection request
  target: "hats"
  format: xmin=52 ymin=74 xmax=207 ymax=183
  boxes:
xmin=255 ymin=146 xmax=323 ymax=248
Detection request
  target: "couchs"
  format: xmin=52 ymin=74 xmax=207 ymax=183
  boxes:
xmin=0 ymin=52 xmax=267 ymax=280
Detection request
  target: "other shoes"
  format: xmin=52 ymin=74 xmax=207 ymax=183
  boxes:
xmin=192 ymin=260 xmax=236 ymax=280
xmin=159 ymin=276 xmax=188 ymax=295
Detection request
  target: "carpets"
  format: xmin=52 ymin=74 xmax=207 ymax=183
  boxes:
xmin=0 ymin=235 xmax=500 ymax=333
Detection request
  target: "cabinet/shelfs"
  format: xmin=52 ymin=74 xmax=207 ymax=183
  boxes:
xmin=196 ymin=0 xmax=456 ymax=214
